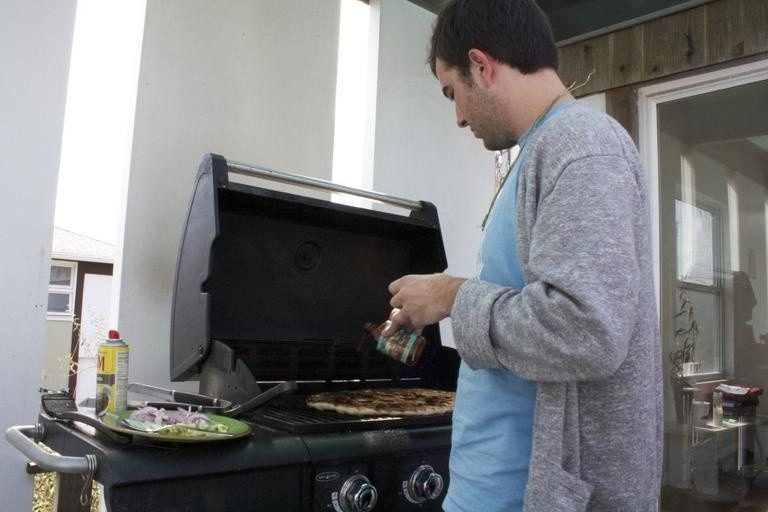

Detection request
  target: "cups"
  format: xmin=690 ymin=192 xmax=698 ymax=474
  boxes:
xmin=692 ymin=402 xmax=709 ymax=427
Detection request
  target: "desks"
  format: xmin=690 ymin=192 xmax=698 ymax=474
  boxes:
xmin=664 ymin=415 xmax=768 ymax=494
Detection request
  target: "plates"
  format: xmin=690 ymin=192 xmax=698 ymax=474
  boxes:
xmin=97 ymin=408 xmax=251 ymax=444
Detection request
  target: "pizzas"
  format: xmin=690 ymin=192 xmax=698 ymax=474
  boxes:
xmin=306 ymin=388 xmax=457 ymax=417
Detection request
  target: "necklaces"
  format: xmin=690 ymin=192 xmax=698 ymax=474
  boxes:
xmin=482 ymin=88 xmax=568 ymax=230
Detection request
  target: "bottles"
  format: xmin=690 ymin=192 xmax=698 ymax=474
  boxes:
xmin=363 ymin=320 xmax=426 ymax=366
xmin=96 ymin=331 xmax=128 ymax=416
xmin=712 ymin=393 xmax=724 ymax=426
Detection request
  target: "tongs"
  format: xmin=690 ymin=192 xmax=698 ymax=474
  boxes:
xmin=79 ymin=381 xmax=232 ymax=414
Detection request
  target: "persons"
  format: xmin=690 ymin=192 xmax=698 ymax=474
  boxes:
xmin=381 ymin=0 xmax=664 ymax=512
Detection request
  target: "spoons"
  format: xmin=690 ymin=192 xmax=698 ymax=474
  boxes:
xmin=117 ymin=418 xmax=237 ymax=439
